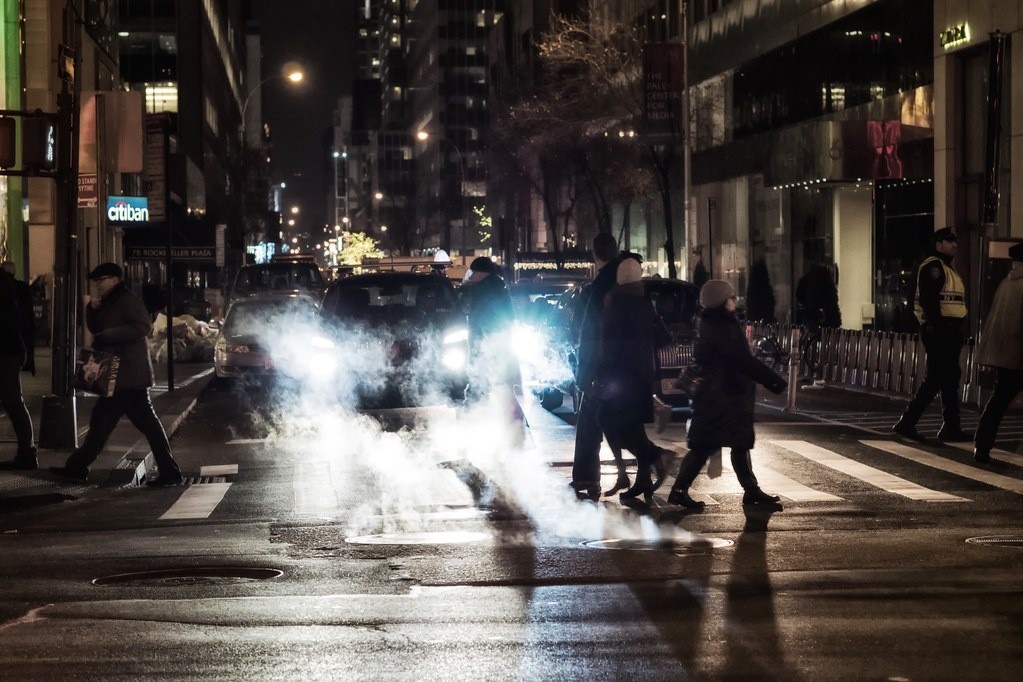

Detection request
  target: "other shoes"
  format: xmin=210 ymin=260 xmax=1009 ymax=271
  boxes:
xmin=667 ymin=491 xmax=705 ymax=508
xmin=937 ymin=427 xmax=975 ymax=442
xmin=565 ymin=482 xmax=601 ymax=497
xmin=50 ymin=466 xmax=91 ymax=480
xmin=974 ymin=447 xmax=990 ymax=459
xmin=743 ymin=489 xmax=780 ymax=503
xmin=0 ymin=458 xmax=39 ymax=471
xmin=647 ymin=446 xmax=676 ymax=492
xmin=893 ymin=418 xmax=929 ymax=443
xmin=146 ymin=474 xmax=183 ymax=486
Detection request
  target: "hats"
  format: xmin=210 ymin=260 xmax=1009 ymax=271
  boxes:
xmin=87 ymin=262 xmax=122 ymax=278
xmin=1008 ymin=243 xmax=1023 ymax=261
xmin=617 ymin=258 xmax=641 ymax=281
xmin=932 ymin=227 xmax=960 ymax=242
xmin=700 ymin=281 xmax=733 ymax=310
xmin=432 ymin=264 xmax=446 ymax=268
xmin=470 ymin=256 xmax=497 ymax=273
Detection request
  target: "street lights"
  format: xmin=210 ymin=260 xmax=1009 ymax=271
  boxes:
xmin=416 ymin=131 xmax=468 ymax=261
xmin=236 ymin=68 xmax=305 ymax=261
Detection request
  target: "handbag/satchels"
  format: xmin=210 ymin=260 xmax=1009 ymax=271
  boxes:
xmin=73 ymin=339 xmax=120 ymax=397
xmin=672 ymin=366 xmax=708 ymax=399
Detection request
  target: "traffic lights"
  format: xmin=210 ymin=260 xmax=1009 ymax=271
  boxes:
xmin=26 ymin=113 xmax=59 ymax=169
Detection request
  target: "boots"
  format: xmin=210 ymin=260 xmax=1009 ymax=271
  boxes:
xmin=620 ymin=469 xmax=653 ymax=499
xmin=605 ymin=465 xmax=630 ymax=496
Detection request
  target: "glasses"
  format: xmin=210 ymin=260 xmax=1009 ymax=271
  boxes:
xmin=93 ymin=277 xmax=113 ymax=283
xmin=441 ymin=268 xmax=445 ymax=270
xmin=728 ymin=292 xmax=737 ymax=303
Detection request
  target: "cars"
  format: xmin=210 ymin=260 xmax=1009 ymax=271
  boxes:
xmin=500 ymin=280 xmax=710 ymax=414
xmin=317 ymin=270 xmax=473 ymax=409
xmin=213 ymin=294 xmax=326 ymax=399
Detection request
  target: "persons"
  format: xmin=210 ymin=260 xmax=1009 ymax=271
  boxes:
xmin=0 ymin=260 xmax=182 ymax=487
xmin=971 ymin=243 xmax=1023 ymax=461
xmin=563 ymin=234 xmax=670 ymax=501
xmin=893 ymin=227 xmax=975 ymax=443
xmin=667 ymin=280 xmax=787 ymax=508
xmin=455 ymin=256 xmax=531 ymax=435
